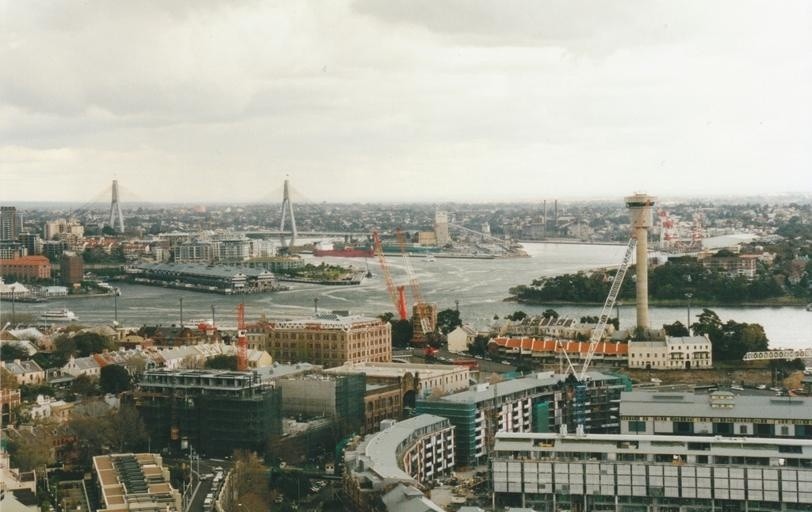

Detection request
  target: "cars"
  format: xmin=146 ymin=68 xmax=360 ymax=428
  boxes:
xmin=406 ymin=345 xmax=513 ymax=365
xmin=198 ymin=465 xmax=224 ymax=511
xmin=732 ymin=384 xmax=789 ymax=397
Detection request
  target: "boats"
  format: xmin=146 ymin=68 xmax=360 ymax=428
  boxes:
xmin=39 ymin=306 xmax=81 ymax=322
